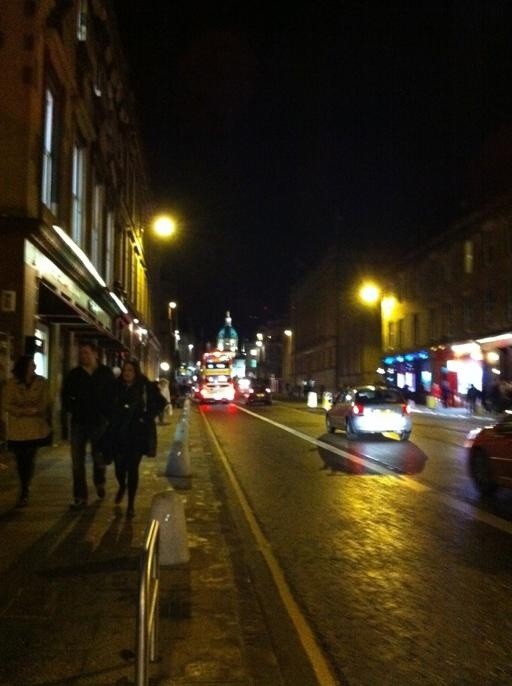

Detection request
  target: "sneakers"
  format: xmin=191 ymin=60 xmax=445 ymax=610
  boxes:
xmin=16 ymin=484 xmax=136 ymax=518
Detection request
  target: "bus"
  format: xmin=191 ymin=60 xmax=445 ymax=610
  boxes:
xmin=198 ymin=350 xmax=234 ymax=403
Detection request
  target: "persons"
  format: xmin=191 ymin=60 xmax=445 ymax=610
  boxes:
xmin=402 ymin=384 xmax=412 ymax=394
xmin=440 ymin=374 xmax=452 ymax=409
xmin=0 ymin=353 xmax=54 ymax=507
xmin=114 ymin=356 xmax=169 ymax=517
xmin=59 ymin=341 xmax=117 ymax=518
xmin=156 ymin=377 xmax=173 ymax=425
xmin=416 ymin=380 xmax=426 ymax=404
xmin=466 ymin=376 xmax=512 ymax=416
xmin=431 ymin=381 xmax=441 ymax=409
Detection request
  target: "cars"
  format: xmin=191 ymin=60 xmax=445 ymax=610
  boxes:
xmin=247 ymin=386 xmax=273 ymax=404
xmin=326 ymin=385 xmax=411 ymax=442
xmin=464 ymin=409 xmax=512 ymax=494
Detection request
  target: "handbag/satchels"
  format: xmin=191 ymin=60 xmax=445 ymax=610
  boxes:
xmin=95 ymin=430 xmax=114 ymax=466
xmin=145 ymin=418 xmax=157 ymax=457
